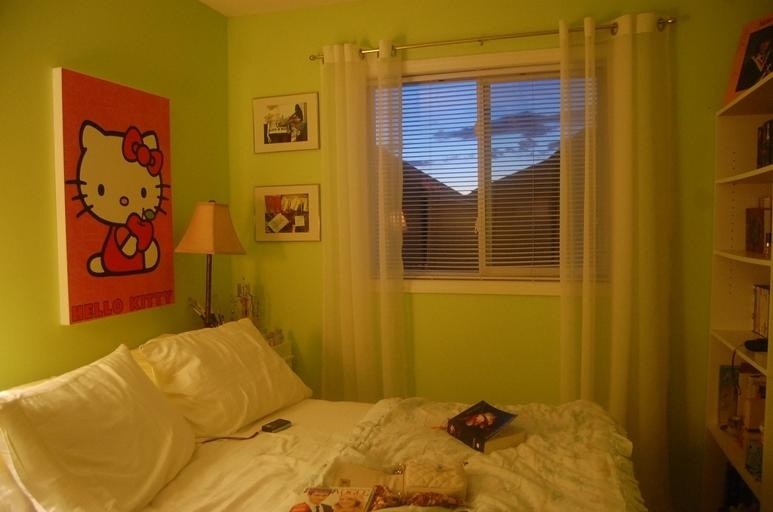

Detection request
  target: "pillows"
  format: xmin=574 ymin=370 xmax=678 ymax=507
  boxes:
xmin=139 ymin=317 xmax=314 ymax=443
xmin=2 ymin=344 xmax=200 ymax=511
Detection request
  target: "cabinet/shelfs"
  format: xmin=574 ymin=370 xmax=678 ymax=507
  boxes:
xmin=707 ymin=79 xmax=771 ymax=512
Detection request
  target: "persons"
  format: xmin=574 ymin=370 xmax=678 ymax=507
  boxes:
xmin=289 ymin=486 xmax=363 ymax=512
xmin=736 ymin=38 xmax=773 ymax=90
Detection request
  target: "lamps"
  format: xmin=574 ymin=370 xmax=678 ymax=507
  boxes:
xmin=175 ymin=202 xmax=247 ymax=325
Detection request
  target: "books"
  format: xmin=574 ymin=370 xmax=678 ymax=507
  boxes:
xmin=289 ymin=486 xmax=374 ymax=512
xmin=745 ymin=196 xmax=773 ymax=258
xmin=447 ymin=400 xmax=526 ymax=455
xmin=718 ymin=365 xmax=767 ymax=429
xmin=752 ymin=284 xmax=770 ymax=338
xmin=757 ymin=120 xmax=773 ymax=168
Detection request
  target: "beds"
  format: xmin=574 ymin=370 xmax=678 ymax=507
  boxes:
xmin=0 ymin=399 xmax=644 ymax=511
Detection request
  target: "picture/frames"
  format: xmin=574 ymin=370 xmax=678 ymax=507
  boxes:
xmin=251 ymin=93 xmax=319 ymax=153
xmin=253 ymin=184 xmax=321 ymax=242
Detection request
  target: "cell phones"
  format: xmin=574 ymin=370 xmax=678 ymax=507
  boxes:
xmin=262 ymin=418 xmax=292 ymax=434
